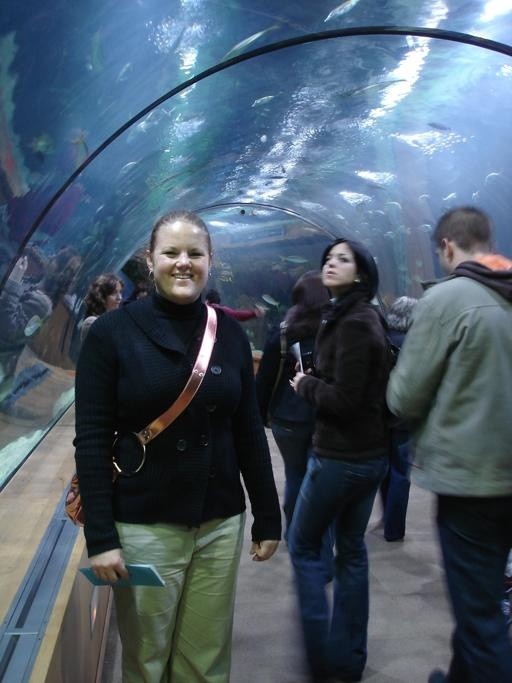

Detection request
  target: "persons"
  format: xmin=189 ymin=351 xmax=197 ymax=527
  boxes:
xmin=384 ymin=205 xmax=510 ymax=683
xmin=1 ymin=240 xmax=54 ymax=412
xmin=69 ymin=208 xmax=281 ymax=683
xmin=67 ymin=245 xmax=424 ymax=547
xmin=287 ymin=233 xmax=393 ymax=683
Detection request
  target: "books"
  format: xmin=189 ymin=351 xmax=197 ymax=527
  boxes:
xmin=76 ymin=562 xmax=165 ymax=590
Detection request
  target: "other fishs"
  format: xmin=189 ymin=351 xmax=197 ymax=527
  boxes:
xmin=83 ymin=64 xmax=94 ymax=73
xmin=70 ymin=78 xmax=505 ymax=345
xmin=26 ymin=130 xmax=54 ymax=154
xmin=23 ymin=314 xmax=48 ymax=336
xmin=323 ymin=0 xmax=360 ymax=23
xmin=222 ymin=26 xmax=279 ymax=60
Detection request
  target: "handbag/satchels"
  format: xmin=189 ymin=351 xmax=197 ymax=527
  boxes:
xmin=263 ymin=377 xmax=281 ymax=426
xmin=64 ymin=460 xmax=123 ymax=526
